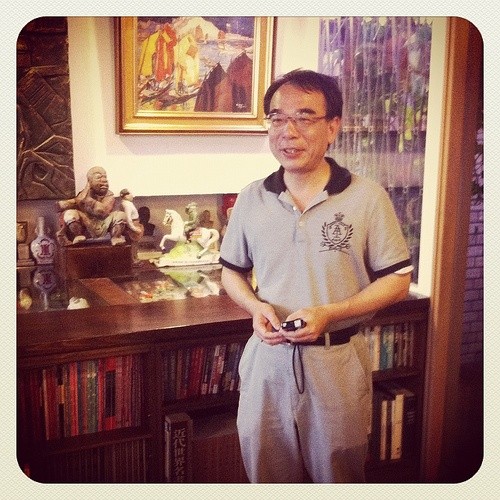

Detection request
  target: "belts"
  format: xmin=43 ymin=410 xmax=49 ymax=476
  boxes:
xmin=286 ymin=322 xmax=359 ymax=345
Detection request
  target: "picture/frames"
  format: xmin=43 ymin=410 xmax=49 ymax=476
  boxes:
xmin=113 ymin=16 xmax=279 ymax=137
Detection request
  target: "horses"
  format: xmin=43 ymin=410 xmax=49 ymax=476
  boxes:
xmin=159 ymin=208 xmax=219 ymax=259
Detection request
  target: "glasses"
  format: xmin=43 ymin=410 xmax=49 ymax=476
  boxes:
xmin=263 ymin=111 xmax=326 ymax=129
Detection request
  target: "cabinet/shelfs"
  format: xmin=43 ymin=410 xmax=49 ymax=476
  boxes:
xmin=16 ymin=239 xmax=430 ymax=484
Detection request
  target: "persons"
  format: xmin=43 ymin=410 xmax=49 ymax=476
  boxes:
xmin=183 ymin=202 xmax=198 ymax=243
xmin=58 ymin=166 xmax=127 ymax=247
xmin=218 ymin=71 xmax=415 ymax=483
xmin=120 ymin=189 xmax=145 ymax=267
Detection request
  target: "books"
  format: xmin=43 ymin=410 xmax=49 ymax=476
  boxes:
xmin=363 ymin=322 xmax=414 ymax=463
xmin=41 ymin=354 xmax=146 ymax=483
xmin=162 ymin=341 xmax=246 ymax=483
